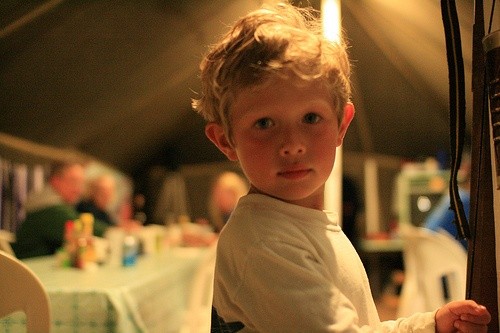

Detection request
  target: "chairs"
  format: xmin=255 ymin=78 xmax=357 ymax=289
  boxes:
xmin=401 ymin=223 xmax=468 ymax=311
xmin=0 ymin=248 xmax=52 ymax=333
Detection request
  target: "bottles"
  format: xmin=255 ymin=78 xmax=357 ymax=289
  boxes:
xmin=75 ymin=213 xmax=96 ymax=269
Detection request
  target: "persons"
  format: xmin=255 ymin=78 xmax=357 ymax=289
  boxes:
xmin=340 ymin=171 xmax=360 ymax=255
xmin=421 ymin=142 xmax=474 ymax=299
xmin=188 ymin=1 xmax=494 ymax=333
xmin=15 ymin=158 xmax=123 ymax=260
xmin=75 ymin=171 xmax=142 ymax=233
xmin=180 ymin=170 xmax=251 ymax=247
xmin=104 ymin=175 xmax=145 ymax=229
xmin=145 ymin=160 xmax=189 ymax=226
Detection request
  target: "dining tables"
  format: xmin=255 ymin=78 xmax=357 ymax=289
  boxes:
xmin=0 ymin=224 xmax=219 ymax=333
